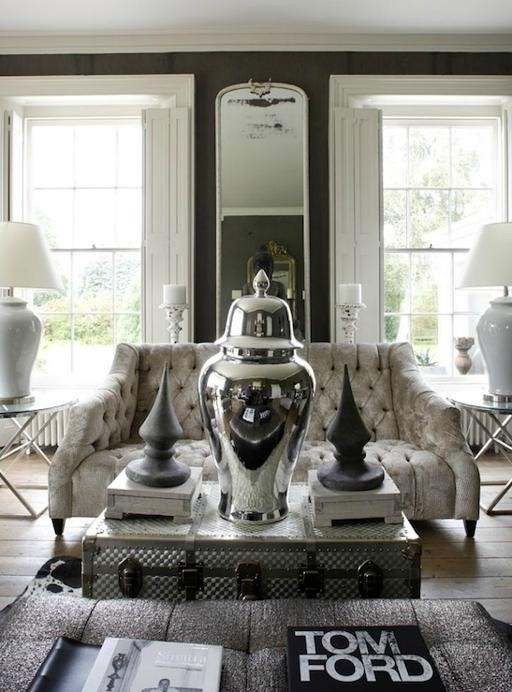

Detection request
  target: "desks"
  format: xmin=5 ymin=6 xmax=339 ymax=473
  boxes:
xmin=447 ymin=394 xmax=512 ymax=515
xmin=0 ymin=395 xmax=79 ymax=518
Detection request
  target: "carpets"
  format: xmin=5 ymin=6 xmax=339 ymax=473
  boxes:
xmin=1 ymin=554 xmax=82 ymax=615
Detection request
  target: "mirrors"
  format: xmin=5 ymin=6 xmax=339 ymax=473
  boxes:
xmin=245 ymin=241 xmax=296 ymax=326
xmin=214 ymin=82 xmax=313 ymax=343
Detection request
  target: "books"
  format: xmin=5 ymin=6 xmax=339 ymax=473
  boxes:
xmin=284 ymin=621 xmax=446 ymax=691
xmin=81 ymin=636 xmax=225 ymax=691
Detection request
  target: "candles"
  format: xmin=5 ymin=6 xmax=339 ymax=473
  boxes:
xmin=163 ymin=285 xmax=186 ymax=304
xmin=338 ymin=283 xmax=362 ymax=303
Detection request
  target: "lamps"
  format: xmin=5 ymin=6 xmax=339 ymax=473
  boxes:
xmin=455 ymin=221 xmax=511 ymax=402
xmin=0 ymin=221 xmax=64 ymax=400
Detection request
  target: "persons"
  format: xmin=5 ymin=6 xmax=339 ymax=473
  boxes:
xmin=150 ymin=677 xmax=179 ymax=691
xmin=240 ymin=243 xmax=300 ymax=331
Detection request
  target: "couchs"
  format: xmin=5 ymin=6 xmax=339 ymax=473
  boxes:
xmin=46 ymin=341 xmax=482 ymax=537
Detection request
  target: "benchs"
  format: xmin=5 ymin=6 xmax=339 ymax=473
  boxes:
xmin=1 ymin=591 xmax=512 ymax=692
